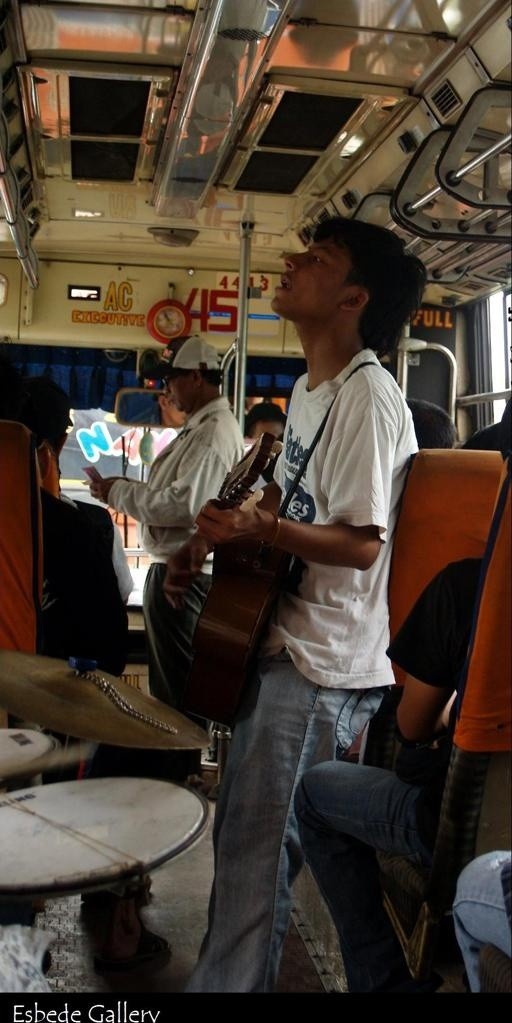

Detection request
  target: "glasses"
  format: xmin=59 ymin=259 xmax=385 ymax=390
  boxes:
xmin=161 ymin=373 xmax=180 ymax=385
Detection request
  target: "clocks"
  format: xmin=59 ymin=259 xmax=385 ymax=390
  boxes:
xmin=147 ymin=298 xmax=192 ymax=345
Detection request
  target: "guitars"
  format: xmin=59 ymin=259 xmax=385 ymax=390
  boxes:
xmin=185 ymin=430 xmax=294 ymax=730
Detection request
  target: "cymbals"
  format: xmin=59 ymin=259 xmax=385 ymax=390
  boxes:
xmin=0 ymin=644 xmax=213 ymax=750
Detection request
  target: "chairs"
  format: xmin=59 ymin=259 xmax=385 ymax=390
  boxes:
xmin=0 ymin=420 xmax=43 ymax=655
xmin=377 ymin=457 xmax=511 ymax=983
xmin=479 ymin=937 xmax=511 ymax=994
xmin=37 ymin=440 xmax=60 ymax=500
xmin=351 ymin=448 xmax=505 ymax=764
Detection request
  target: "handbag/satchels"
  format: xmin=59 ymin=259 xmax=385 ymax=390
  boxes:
xmin=395 ymin=745 xmax=452 ymax=790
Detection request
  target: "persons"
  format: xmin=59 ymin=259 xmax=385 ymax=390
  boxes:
xmin=1 ymin=220 xmax=511 ymax=995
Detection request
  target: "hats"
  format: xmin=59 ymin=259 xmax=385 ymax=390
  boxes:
xmin=1 ymin=376 xmax=75 ymax=427
xmin=145 ymin=336 xmax=219 ymax=379
